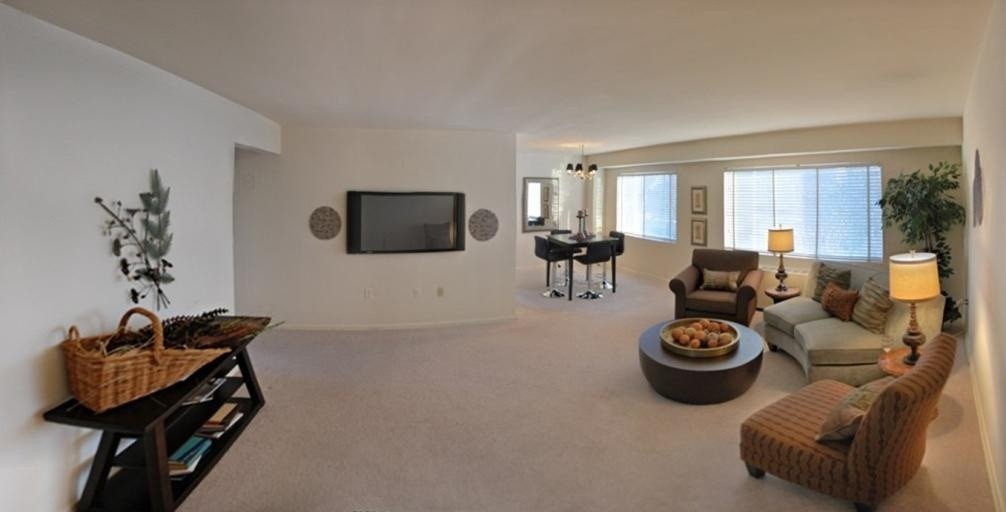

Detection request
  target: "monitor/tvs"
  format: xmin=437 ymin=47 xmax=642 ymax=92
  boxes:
xmin=346 ymin=190 xmax=465 ymax=254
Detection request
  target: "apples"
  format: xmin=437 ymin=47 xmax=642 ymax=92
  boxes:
xmin=673 ymin=318 xmax=733 ymax=350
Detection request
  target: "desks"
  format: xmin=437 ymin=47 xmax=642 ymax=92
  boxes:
xmin=43 ymin=316 xmax=270 ymax=512
xmin=545 ymin=232 xmax=618 ymax=300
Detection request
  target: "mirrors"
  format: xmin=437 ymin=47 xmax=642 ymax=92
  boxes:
xmin=523 ymin=176 xmax=560 ymax=232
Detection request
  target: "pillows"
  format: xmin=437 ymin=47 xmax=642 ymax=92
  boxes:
xmin=699 ymin=267 xmax=740 ymax=292
xmin=851 ymin=276 xmax=893 ymax=335
xmin=815 ymin=376 xmax=892 ymax=445
xmin=821 ymin=283 xmax=858 ymax=322
xmin=812 ymin=262 xmax=851 ymax=303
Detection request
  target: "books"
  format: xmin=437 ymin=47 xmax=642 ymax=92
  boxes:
xmin=163 ymin=376 xmax=246 ymax=482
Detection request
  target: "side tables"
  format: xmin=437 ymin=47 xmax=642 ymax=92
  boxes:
xmin=764 ymin=288 xmax=800 ymax=304
xmin=878 ymin=348 xmax=921 ymax=377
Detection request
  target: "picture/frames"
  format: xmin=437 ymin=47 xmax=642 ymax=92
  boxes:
xmin=691 ymin=186 xmax=706 ymax=214
xmin=691 ymin=220 xmax=706 ymax=246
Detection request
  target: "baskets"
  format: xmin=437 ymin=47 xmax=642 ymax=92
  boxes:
xmin=62 ymin=308 xmax=231 ymax=414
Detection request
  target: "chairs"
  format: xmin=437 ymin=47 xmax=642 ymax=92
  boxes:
xmin=534 ymin=236 xmax=568 ymax=297
xmin=573 ymin=240 xmax=610 ymax=300
xmin=739 ymin=332 xmax=957 ymax=512
xmin=598 ymin=231 xmax=624 ymax=289
xmin=551 ymin=229 xmax=582 ymax=287
xmin=669 ymin=249 xmax=763 ymax=327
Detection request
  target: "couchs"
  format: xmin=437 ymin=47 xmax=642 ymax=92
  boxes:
xmin=763 ymin=261 xmax=946 ymax=387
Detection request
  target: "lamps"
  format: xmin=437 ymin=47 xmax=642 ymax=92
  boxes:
xmin=565 ymin=145 xmax=598 ymax=181
xmin=767 ymin=224 xmax=794 ymax=291
xmin=888 ymin=250 xmax=940 ymax=365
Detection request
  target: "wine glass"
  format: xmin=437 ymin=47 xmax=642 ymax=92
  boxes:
xmin=881 ymin=335 xmax=895 ymax=364
